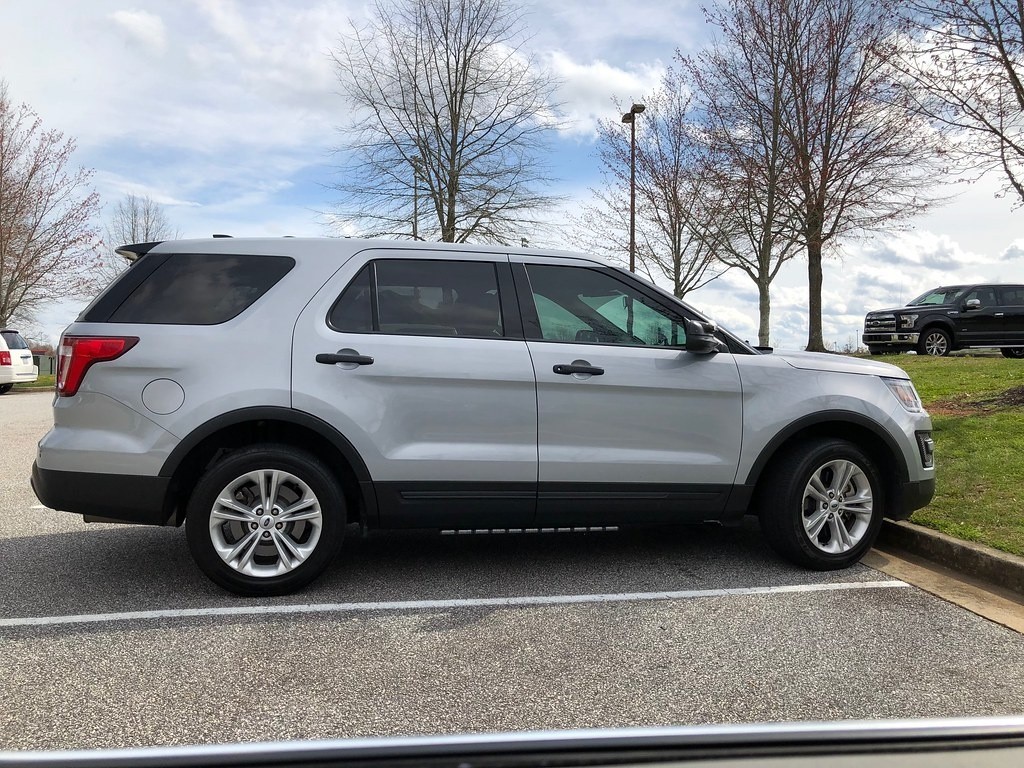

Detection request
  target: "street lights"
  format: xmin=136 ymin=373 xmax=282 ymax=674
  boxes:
xmin=621 ymin=104 xmax=646 ymax=340
xmin=410 ymin=153 xmax=424 ymax=242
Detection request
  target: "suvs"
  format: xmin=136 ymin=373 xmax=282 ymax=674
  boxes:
xmin=39 ymin=233 xmax=938 ymax=596
xmin=1 ymin=328 xmax=39 ymax=394
xmin=863 ymin=283 xmax=1024 ymax=360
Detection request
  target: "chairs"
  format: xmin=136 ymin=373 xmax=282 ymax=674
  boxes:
xmin=354 ymin=285 xmax=504 ymax=335
xmin=946 ymin=295 xmax=1024 ymax=305
